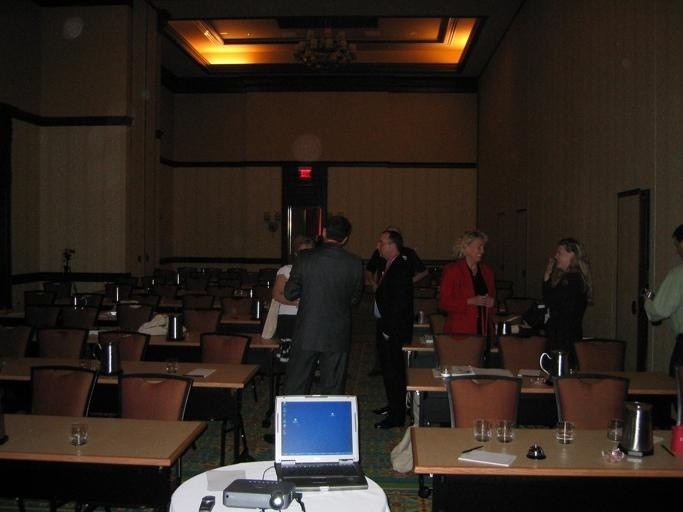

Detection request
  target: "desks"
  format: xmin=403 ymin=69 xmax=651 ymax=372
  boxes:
xmin=163 ymin=460 xmax=391 ymax=512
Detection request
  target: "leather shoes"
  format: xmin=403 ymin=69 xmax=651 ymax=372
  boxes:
xmin=372 ymin=405 xmax=389 ymax=416
xmin=263 ymin=433 xmax=275 ymax=446
xmin=375 ymin=419 xmax=403 ymax=429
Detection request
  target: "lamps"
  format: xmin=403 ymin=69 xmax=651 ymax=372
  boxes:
xmin=286 ymin=23 xmax=362 ymax=72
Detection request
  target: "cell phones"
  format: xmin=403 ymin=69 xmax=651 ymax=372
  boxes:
xmin=198 ymin=495 xmax=216 ymax=512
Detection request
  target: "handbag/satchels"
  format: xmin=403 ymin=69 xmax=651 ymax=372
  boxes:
xmin=262 ymin=296 xmax=281 ymax=340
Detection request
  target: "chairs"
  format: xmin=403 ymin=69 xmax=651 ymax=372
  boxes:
xmin=402 ymin=283 xmax=681 ymax=512
xmin=0 ymin=268 xmax=281 ymax=512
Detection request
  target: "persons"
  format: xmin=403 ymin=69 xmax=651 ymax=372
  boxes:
xmin=437 ymin=230 xmax=496 ymax=337
xmin=643 ymin=225 xmax=683 ymax=377
xmin=262 ymin=215 xmax=428 ymax=443
xmin=542 ymin=237 xmax=592 ymax=371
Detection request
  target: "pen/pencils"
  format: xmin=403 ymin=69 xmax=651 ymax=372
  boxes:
xmin=660 ymin=444 xmax=675 ymax=456
xmin=463 ymin=445 xmax=484 ymax=453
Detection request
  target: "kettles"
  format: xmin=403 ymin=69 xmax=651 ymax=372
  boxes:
xmin=539 ymin=350 xmax=567 ymax=385
xmin=501 ymin=320 xmax=510 ymax=336
xmin=617 ymin=401 xmax=654 ymax=457
xmin=72 ymin=268 xmax=271 ymax=321
xmin=166 ymin=314 xmax=184 ymax=341
xmin=418 ymin=310 xmax=426 ymax=324
xmin=96 ymin=341 xmax=121 ymax=374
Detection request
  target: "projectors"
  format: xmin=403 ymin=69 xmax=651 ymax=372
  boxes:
xmin=222 ymin=478 xmax=296 ymax=511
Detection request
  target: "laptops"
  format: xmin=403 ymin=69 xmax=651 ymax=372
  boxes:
xmin=274 ymin=393 xmax=369 ymax=492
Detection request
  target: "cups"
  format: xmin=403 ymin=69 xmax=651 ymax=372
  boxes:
xmin=608 ymin=418 xmax=623 ymax=440
xmin=472 ymin=416 xmax=514 ymax=442
xmin=672 ymin=425 xmax=683 ymax=455
xmin=165 ymin=360 xmax=178 ymax=375
xmin=439 ymin=366 xmax=452 ymax=383
xmin=71 ymin=420 xmax=88 ymax=446
xmin=556 ymin=420 xmax=576 ymax=444
xmin=568 ymin=368 xmax=576 ymax=376
